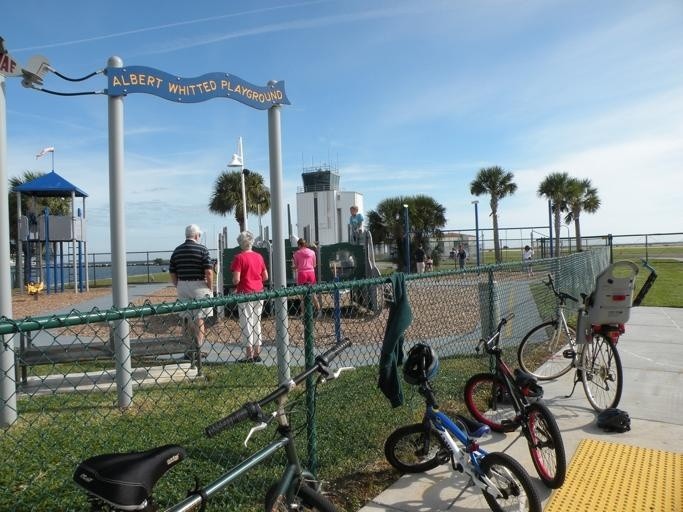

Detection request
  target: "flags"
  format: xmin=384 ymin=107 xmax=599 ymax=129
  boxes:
xmin=34 ymin=143 xmax=55 ymax=160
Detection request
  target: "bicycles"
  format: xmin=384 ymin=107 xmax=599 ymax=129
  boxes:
xmin=73 ymin=337 xmax=353 ymax=512
xmin=517 ymin=259 xmax=640 ymax=413
xmin=464 ymin=314 xmax=566 ymax=490
xmin=383 ymin=345 xmax=542 ymax=512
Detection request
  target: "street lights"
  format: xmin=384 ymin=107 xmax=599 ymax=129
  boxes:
xmin=472 ymin=200 xmax=479 ymax=267
xmin=228 ymin=136 xmax=248 ymax=232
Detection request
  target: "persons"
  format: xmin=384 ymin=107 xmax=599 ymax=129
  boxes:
xmin=413 ymin=242 xmax=426 ymax=275
xmin=521 ymin=244 xmax=534 ymax=278
xmin=451 ymin=247 xmax=457 ymax=260
xmin=168 ymin=224 xmax=214 ymax=360
xmin=348 ymin=204 xmax=364 ymax=243
xmin=291 ymin=237 xmax=321 ymax=320
xmin=455 ymin=244 xmax=466 ymax=270
xmin=425 ymin=256 xmax=433 ymax=272
xmin=228 ymin=230 xmax=270 ymax=364
xmin=430 ymin=250 xmax=441 ymax=272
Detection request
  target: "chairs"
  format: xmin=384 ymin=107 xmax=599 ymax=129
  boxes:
xmin=575 ymin=261 xmax=640 ymax=344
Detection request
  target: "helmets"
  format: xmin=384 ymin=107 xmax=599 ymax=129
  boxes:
xmin=403 ymin=347 xmax=439 ymax=384
xmin=598 ymin=407 xmax=631 ymax=434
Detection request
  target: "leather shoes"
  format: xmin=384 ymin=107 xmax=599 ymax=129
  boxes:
xmin=255 ymin=356 xmax=262 ymax=362
xmin=184 ymin=351 xmax=209 ymax=360
xmin=238 ymin=358 xmax=254 ymax=363
